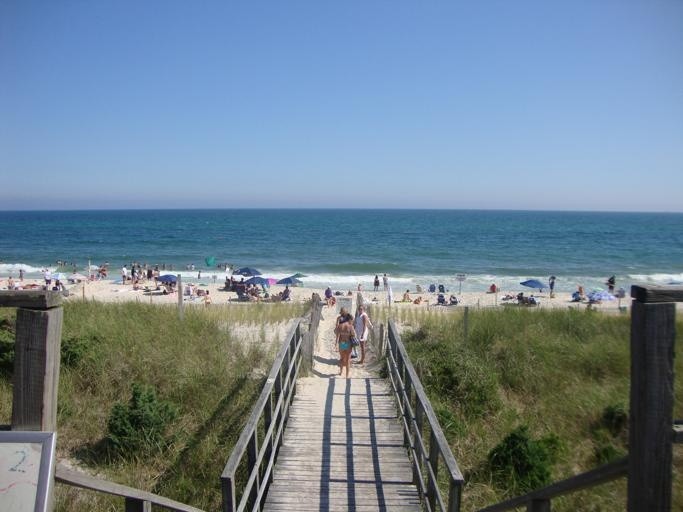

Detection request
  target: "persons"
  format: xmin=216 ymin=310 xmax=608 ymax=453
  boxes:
xmin=121 ymin=261 xmax=176 ymax=293
xmin=334 ymin=305 xmax=374 ymax=378
xmin=572 ymin=275 xmax=625 ymax=301
xmin=184 ymin=262 xmax=290 ymax=304
xmin=404 ymin=285 xmax=458 ymax=305
xmin=490 ymin=283 xmax=497 ymax=292
xmin=516 ymin=276 xmax=556 ymax=305
xmin=358 ymin=273 xmax=388 ymax=291
xmin=312 ymin=286 xmax=336 ymax=305
xmin=7 ymin=260 xmax=107 ymax=290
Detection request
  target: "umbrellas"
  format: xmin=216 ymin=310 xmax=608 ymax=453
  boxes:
xmin=276 ymin=277 xmax=303 ymax=284
xmin=291 ymin=272 xmax=307 ymax=277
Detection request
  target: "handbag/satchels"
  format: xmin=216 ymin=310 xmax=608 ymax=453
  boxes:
xmin=350 ymin=337 xmax=359 ymax=346
xmin=367 ymin=320 xmax=373 ymax=329
